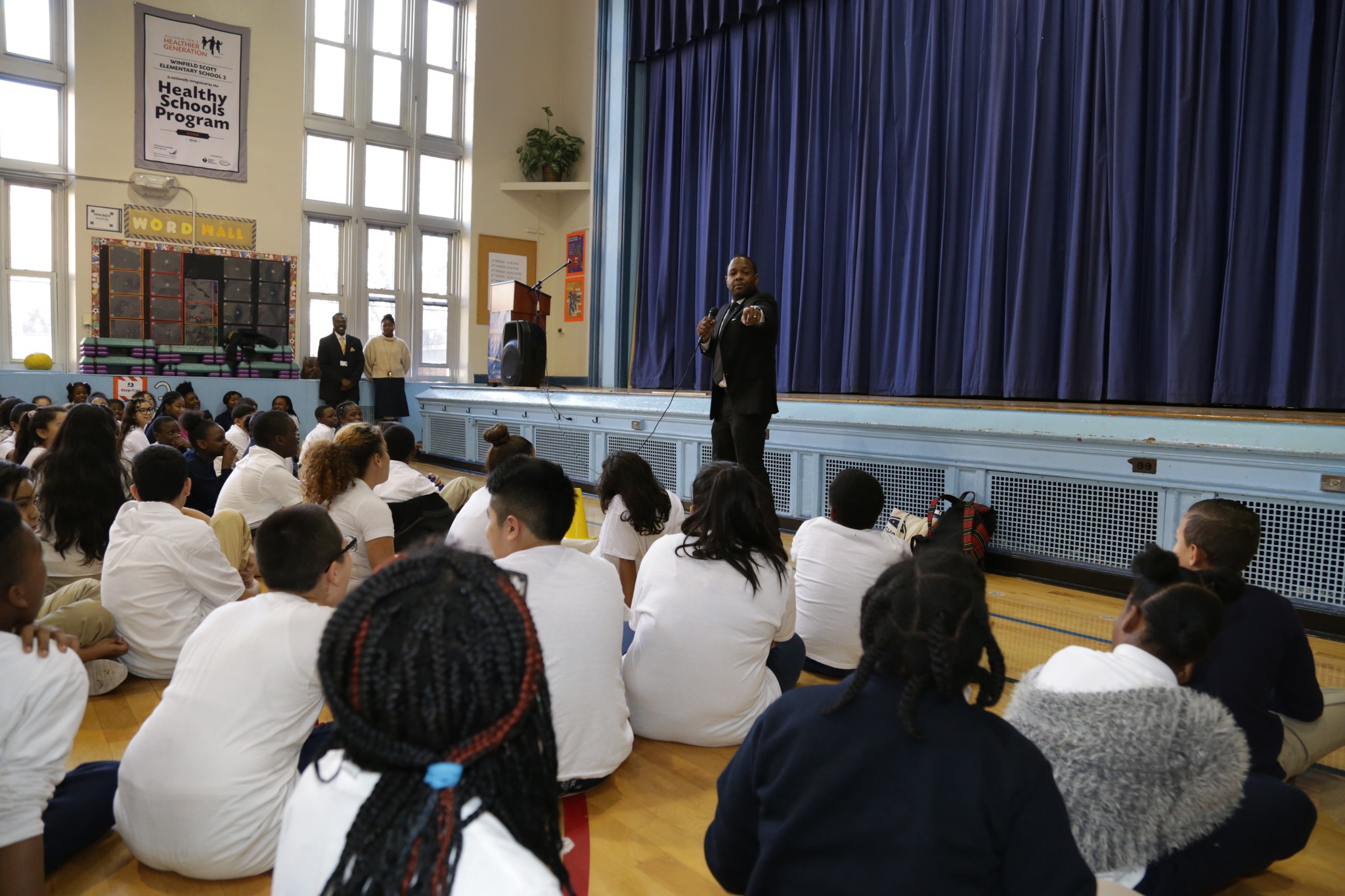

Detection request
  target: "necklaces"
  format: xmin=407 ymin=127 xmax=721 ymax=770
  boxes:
xmin=382 ymin=338 xmax=393 ymax=377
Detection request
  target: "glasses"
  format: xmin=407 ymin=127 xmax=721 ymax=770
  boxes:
xmin=323 ymin=535 xmax=358 ymax=573
xmin=134 ymin=407 xmax=155 ymax=415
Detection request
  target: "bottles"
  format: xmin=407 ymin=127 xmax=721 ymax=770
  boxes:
xmin=428 ymin=476 xmax=444 ymax=491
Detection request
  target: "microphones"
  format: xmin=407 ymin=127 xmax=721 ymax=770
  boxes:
xmin=697 ymin=307 xmax=719 ymax=346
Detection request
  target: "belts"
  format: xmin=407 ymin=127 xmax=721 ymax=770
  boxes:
xmin=717 ymin=386 xmax=727 ymax=393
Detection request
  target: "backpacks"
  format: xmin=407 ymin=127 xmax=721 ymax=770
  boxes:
xmin=910 ymin=490 xmax=998 ymax=563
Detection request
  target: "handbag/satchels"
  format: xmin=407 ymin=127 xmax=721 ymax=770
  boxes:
xmin=882 ymin=506 xmax=936 ymax=547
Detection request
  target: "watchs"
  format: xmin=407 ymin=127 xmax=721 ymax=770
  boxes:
xmin=750 ymin=305 xmax=761 ymax=312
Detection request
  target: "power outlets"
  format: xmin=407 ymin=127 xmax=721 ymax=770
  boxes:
xmin=569 ymin=416 xmax=576 ymax=423
xmin=1132 ymin=457 xmax=1157 ymax=474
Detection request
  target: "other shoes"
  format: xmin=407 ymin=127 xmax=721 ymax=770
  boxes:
xmin=83 ymin=658 xmax=127 ymax=697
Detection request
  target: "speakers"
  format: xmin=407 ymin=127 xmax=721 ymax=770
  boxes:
xmin=500 ymin=320 xmax=546 ymax=386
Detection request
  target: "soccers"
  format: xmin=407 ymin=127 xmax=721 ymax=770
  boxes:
xmin=23 ymin=352 xmax=52 ymax=370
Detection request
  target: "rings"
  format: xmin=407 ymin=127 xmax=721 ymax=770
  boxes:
xmin=753 ymin=315 xmax=757 ymax=318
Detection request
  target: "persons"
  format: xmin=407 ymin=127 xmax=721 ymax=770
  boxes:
xmin=317 ymin=312 xmax=365 ymax=408
xmin=361 ymin=314 xmax=412 ymax=422
xmin=696 ymin=255 xmax=788 ymax=566
xmin=0 ymin=378 xmax=1345 ymax=896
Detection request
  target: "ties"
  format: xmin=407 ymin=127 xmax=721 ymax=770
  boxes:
xmin=713 ymin=302 xmax=738 ymax=385
xmin=340 ymin=337 xmax=346 ymax=356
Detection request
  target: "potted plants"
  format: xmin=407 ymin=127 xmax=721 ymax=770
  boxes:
xmin=515 ymin=107 xmax=584 ymax=182
